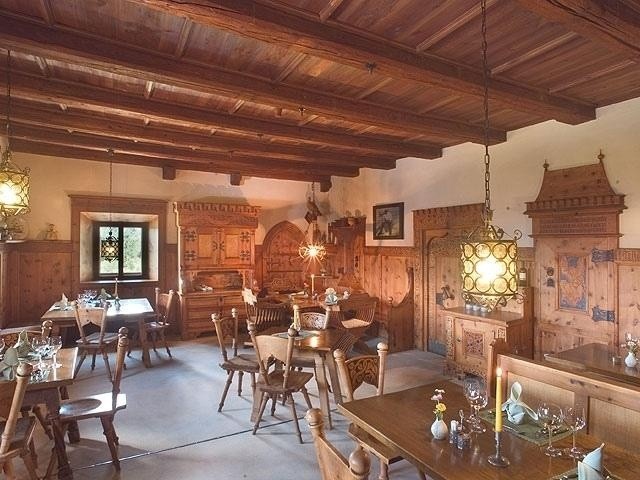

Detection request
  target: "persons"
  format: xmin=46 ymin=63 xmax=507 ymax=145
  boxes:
xmin=378 ymin=209 xmax=393 ymax=236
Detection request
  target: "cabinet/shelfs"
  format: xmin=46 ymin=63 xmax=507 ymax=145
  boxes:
xmin=184 ymin=292 xmax=246 ymax=340
xmin=0 ymin=241 xmax=72 ymax=329
xmin=181 ymin=227 xmax=253 ymax=268
xmin=440 ymin=248 xmax=534 ymax=384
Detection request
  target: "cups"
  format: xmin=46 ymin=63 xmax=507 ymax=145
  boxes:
xmin=31 ymin=335 xmax=49 ymax=369
xmin=84 ymin=294 xmax=90 ymax=307
xmin=47 ymin=335 xmax=63 ymax=371
xmin=463 ymin=379 xmax=481 ymax=424
xmin=77 ymin=293 xmax=85 ymax=309
xmin=83 ymin=289 xmax=92 ymax=298
xmin=561 ymin=405 xmax=588 ymax=461
xmin=469 ymin=389 xmax=489 ymax=434
xmin=90 ymin=290 xmax=98 ymax=307
xmin=536 ymin=400 xmax=565 ymax=457
xmin=0 ymin=338 xmax=5 ymax=359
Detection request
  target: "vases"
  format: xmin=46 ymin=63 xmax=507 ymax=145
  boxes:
xmin=625 ymin=352 xmax=637 ymax=367
xmin=431 ymin=421 xmax=448 ymax=439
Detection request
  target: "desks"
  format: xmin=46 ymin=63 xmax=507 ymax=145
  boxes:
xmin=544 ymin=343 xmax=640 ymax=388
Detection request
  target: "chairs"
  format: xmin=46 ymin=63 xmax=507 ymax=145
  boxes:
xmin=127 ymin=288 xmax=175 ymax=357
xmin=334 ymin=343 xmax=427 ymax=480
xmin=71 ymin=301 xmax=127 ymax=382
xmin=211 ymin=308 xmax=259 ymax=412
xmin=247 ymin=325 xmax=313 ymax=444
xmin=305 ymin=409 xmax=371 ymax=480
xmin=44 ymin=327 xmax=129 ymax=472
xmin=0 ymin=363 xmax=38 ymax=480
xmin=290 ymin=305 xmax=332 ymax=393
xmin=330 ymin=298 xmax=377 ymax=353
xmin=245 ymin=299 xmax=284 ymax=331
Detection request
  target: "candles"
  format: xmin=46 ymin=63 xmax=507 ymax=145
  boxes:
xmin=293 ymin=305 xmax=298 ymax=330
xmin=115 ymin=278 xmax=119 ymax=298
xmin=488 ymin=367 xmax=510 ymax=468
xmin=311 ymin=274 xmax=314 ymax=296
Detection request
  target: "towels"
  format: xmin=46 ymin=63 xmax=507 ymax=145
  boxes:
xmin=323 ymin=286 xmax=337 ymax=296
xmin=59 ymin=294 xmax=69 ymax=311
xmin=324 ymin=294 xmax=338 ymax=305
xmin=499 ymin=381 xmax=540 ymax=426
xmin=576 ymin=442 xmax=607 ymax=479
xmin=0 ymin=347 xmax=23 ymax=382
xmin=11 ymin=329 xmax=31 ymax=358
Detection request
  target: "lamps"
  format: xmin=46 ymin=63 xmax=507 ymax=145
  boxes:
xmin=101 ymin=150 xmax=122 ymax=262
xmin=0 ymin=50 xmax=31 ymax=244
xmin=302 ymin=179 xmax=323 ymax=260
xmin=458 ymin=0 xmax=523 ymax=313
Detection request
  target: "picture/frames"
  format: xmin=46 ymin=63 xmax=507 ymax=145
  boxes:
xmin=373 ymin=203 xmax=404 ymax=239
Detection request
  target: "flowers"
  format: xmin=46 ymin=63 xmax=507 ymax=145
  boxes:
xmin=620 ymin=332 xmax=640 ymax=353
xmin=430 ymin=388 xmax=447 ymax=421
